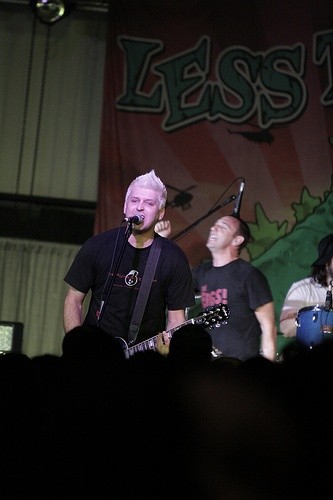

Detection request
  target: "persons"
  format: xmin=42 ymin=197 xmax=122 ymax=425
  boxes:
xmin=0 ymin=322 xmax=333 ymax=500
xmin=63 ymin=169 xmax=196 ymax=356
xmin=154 ymin=215 xmax=277 ymax=360
xmin=279 ymin=233 xmax=333 ymax=338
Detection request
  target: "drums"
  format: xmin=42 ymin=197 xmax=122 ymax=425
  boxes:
xmin=293 ymin=302 xmax=333 ymax=349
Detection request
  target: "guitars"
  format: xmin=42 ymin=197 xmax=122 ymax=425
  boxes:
xmin=115 ymin=303 xmax=231 ymax=361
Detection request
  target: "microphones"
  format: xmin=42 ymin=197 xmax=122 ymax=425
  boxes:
xmin=233 ymin=180 xmax=245 ymax=217
xmin=123 ymin=215 xmax=142 ymax=225
